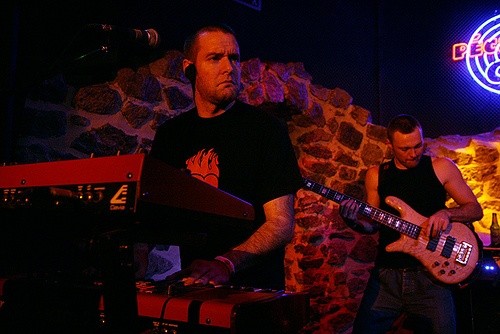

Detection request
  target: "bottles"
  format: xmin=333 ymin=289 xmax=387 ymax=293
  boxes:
xmin=490 ymin=213 xmax=500 ymax=247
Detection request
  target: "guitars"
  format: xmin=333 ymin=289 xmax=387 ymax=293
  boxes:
xmin=301 ymin=177 xmax=479 ymax=283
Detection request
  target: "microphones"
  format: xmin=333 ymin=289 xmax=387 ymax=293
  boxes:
xmin=120 ymin=28 xmax=158 ymax=45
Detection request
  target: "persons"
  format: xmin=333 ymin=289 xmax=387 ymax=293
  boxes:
xmin=338 ymin=114 xmax=483 ymax=334
xmin=133 ymin=23 xmax=303 ymax=287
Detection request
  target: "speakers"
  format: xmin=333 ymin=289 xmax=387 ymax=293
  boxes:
xmin=454 ymin=286 xmax=500 ymax=334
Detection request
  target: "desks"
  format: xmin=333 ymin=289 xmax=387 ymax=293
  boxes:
xmin=472 ymin=247 xmax=500 ymax=281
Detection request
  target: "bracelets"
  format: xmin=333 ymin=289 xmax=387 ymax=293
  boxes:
xmin=214 ymin=255 xmax=236 ymax=274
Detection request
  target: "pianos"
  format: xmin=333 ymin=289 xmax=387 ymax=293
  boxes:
xmin=0 ymin=143 xmax=309 ymax=334
